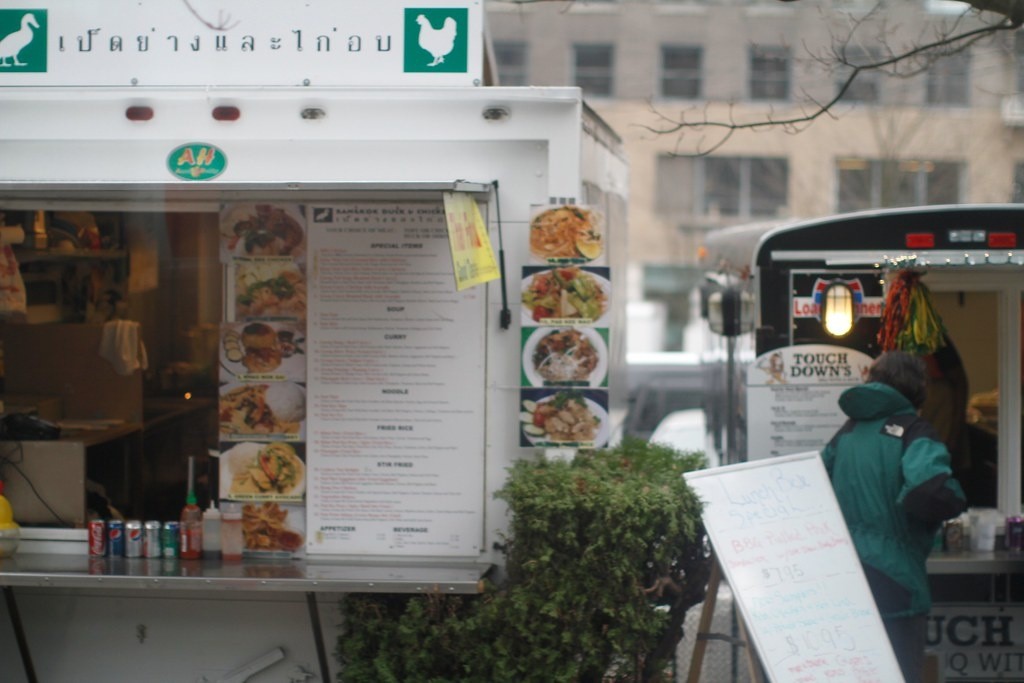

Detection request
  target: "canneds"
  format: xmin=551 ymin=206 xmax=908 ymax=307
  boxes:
xmin=106 ymin=520 xmax=124 ymax=558
xmin=161 ymin=521 xmax=180 ymax=560
xmin=1005 ymin=514 xmax=1024 ymax=551
xmin=88 ymin=519 xmax=106 ymax=556
xmin=143 ymin=520 xmax=163 ymax=558
xmin=124 ymin=520 xmax=144 ymax=558
xmin=944 ymin=518 xmax=963 ymax=551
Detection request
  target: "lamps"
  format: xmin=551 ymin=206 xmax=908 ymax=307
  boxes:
xmin=821 ymin=281 xmax=855 ymax=339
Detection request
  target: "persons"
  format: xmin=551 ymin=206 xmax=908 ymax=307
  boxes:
xmin=821 ymin=348 xmax=968 ymax=683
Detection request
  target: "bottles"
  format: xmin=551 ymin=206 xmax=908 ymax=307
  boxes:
xmin=221 ymin=504 xmax=243 ymax=560
xmin=201 ymin=500 xmax=221 ymax=559
xmin=181 ymin=490 xmax=201 ymax=559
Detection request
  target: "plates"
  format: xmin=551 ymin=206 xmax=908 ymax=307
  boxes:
xmin=219 ymin=383 xmax=306 ymax=442
xmin=219 ymin=321 xmax=306 ymax=379
xmin=523 ymin=395 xmax=610 ymax=449
xmin=521 ymin=268 xmax=611 ymax=328
xmin=522 ymin=204 xmax=607 ymax=268
xmin=521 ymin=327 xmax=608 ymax=389
xmin=220 ymin=450 xmax=306 ymax=500
